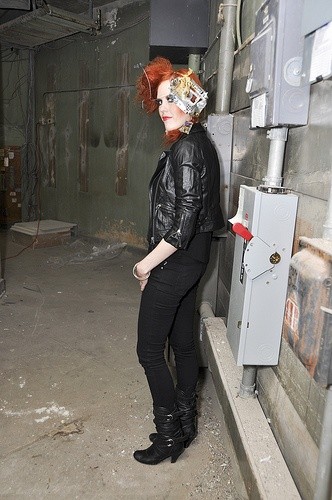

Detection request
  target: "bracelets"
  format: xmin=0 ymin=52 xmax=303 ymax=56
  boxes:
xmin=133 ymin=263 xmax=151 ymax=280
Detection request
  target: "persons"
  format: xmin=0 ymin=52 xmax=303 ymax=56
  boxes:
xmin=133 ymin=59 xmax=225 ymax=465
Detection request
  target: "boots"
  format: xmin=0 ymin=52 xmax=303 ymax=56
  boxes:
xmin=133 ymin=402 xmax=183 ymax=465
xmin=149 ymin=385 xmax=199 ymax=448
